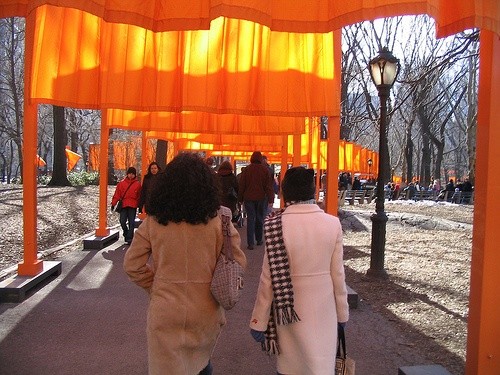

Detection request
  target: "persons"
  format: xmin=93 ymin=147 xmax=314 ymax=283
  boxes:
xmin=237 ymin=150 xmax=276 ymax=250
xmin=249 ymin=167 xmax=351 ymax=375
xmin=110 ymin=167 xmax=143 ymax=246
xmin=123 ymin=150 xmax=249 ymax=375
xmin=233 ymin=165 xmax=474 ymax=202
xmin=139 ymin=161 xmax=160 ymax=221
xmin=215 ymin=159 xmax=239 ymax=226
xmin=204 ymin=157 xmax=215 ymax=170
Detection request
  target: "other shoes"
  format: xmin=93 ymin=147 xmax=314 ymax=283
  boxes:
xmin=247 ymin=240 xmax=263 ymax=250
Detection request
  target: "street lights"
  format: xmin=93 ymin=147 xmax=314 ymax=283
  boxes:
xmin=356 ymin=46 xmax=403 ymax=282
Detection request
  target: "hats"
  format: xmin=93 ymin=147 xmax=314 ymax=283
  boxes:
xmin=281 ymin=166 xmax=316 ymax=201
xmin=127 ymin=167 xmax=136 ymax=177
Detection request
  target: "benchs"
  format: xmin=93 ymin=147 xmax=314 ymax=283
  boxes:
xmin=408 ymin=189 xmax=436 ymax=200
xmin=342 ymin=190 xmax=367 ymax=204
xmin=443 ymin=190 xmax=474 ymax=203
xmin=369 ymin=190 xmax=395 ymax=201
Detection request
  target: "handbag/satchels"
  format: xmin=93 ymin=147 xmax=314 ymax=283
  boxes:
xmin=335 ymin=323 xmax=355 ymax=375
xmin=114 ymin=200 xmax=122 ymax=212
xmin=209 ymin=205 xmax=245 ymax=310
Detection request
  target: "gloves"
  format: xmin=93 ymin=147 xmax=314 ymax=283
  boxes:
xmin=250 ymin=329 xmax=265 ymax=343
xmin=111 ymin=206 xmax=114 ymax=211
